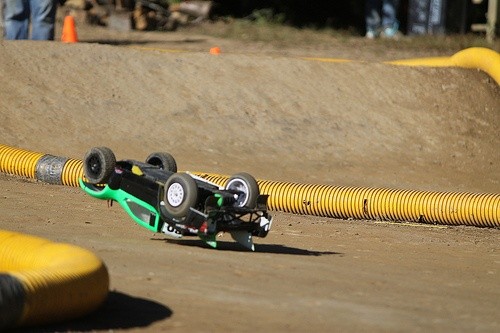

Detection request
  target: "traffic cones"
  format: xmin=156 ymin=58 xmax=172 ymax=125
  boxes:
xmin=60 ymin=16 xmax=78 ymax=44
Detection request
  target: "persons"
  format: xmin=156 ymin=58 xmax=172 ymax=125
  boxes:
xmin=4 ymin=0 xmax=57 ymax=40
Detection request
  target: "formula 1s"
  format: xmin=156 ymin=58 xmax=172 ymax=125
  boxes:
xmin=77 ymin=147 xmax=274 ymax=252
